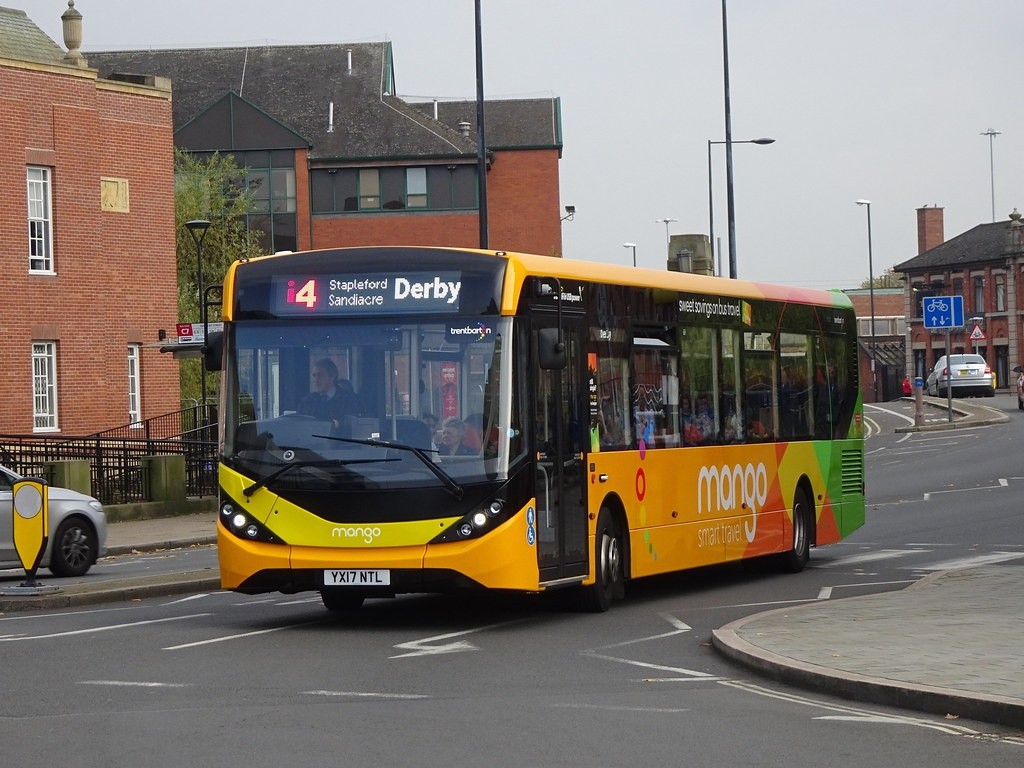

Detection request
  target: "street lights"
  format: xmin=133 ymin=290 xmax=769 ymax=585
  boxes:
xmin=856 ymin=199 xmax=878 ymax=403
xmin=623 ymin=243 xmax=637 ymax=267
xmin=708 ymin=137 xmax=776 ymax=259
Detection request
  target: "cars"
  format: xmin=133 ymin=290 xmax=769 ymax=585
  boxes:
xmin=925 ymin=353 xmax=994 ymax=398
xmin=0 ymin=464 xmax=108 ymax=576
xmin=1013 ymin=365 xmax=1024 ymax=409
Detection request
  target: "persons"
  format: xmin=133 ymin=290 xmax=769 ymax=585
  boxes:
xmin=599 ymin=386 xmax=774 ymax=443
xmin=336 ymin=378 xmax=353 ymax=394
xmin=398 ymin=391 xmax=409 ymax=414
xmin=416 ymin=412 xmax=498 ymax=462
xmin=297 ymin=357 xmax=376 ymax=451
xmin=901 ymin=374 xmax=912 ymax=397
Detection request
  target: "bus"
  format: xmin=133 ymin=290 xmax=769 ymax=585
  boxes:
xmin=216 ymin=245 xmax=865 ymax=614
